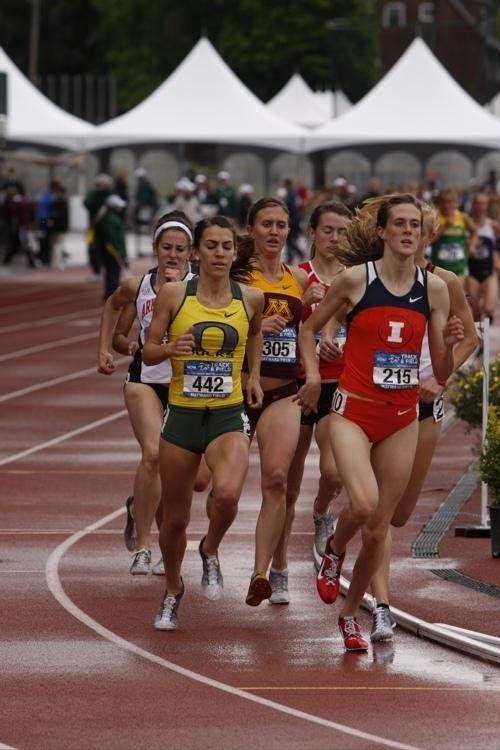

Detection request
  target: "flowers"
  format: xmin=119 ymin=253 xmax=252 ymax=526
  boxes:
xmin=447 ymin=352 xmax=500 ymax=506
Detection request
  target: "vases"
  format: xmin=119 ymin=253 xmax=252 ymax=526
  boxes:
xmin=487 ymin=506 xmax=500 ymax=559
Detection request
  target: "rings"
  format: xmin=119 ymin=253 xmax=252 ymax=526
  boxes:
xmin=326 ymin=348 xmax=330 ymax=352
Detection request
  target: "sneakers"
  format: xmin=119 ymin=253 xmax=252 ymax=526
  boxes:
xmin=269 ymin=567 xmax=289 ymax=604
xmin=370 ymin=598 xmax=394 ymax=642
xmin=199 ymin=536 xmax=223 ymax=601
xmin=316 ymin=534 xmax=345 ymax=604
xmin=129 ymin=549 xmax=165 ymax=575
xmin=245 ymin=569 xmax=272 ymax=606
xmin=154 ymin=577 xmax=184 ymax=630
xmin=124 ymin=496 xmax=136 ymax=551
xmin=338 ymin=614 xmax=368 ymax=651
xmin=312 ymin=496 xmax=334 ymax=557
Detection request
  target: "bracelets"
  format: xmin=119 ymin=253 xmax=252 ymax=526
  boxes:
xmin=128 ymin=340 xmax=135 ymax=355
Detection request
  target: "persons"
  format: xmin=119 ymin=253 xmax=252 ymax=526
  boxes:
xmin=374 ymin=646 xmax=398 ymax=667
xmin=83 ymin=167 xmax=160 ymax=302
xmin=110 ymin=211 xmax=210 ymax=551
xmin=167 ymin=170 xmax=252 ymax=234
xmin=218 ymin=204 xmax=254 ymax=272
xmin=348 ymin=191 xmax=479 ymax=645
xmin=291 ymin=192 xmax=465 ymax=652
xmin=266 ymin=201 xmax=356 ymax=604
xmin=276 ymin=175 xmax=499 ymax=354
xmin=200 ymin=196 xmax=311 ymax=607
xmin=94 ymin=209 xmax=204 ymax=579
xmin=1 ymin=165 xmax=69 ymax=268
xmin=141 ymin=213 xmax=266 ymax=635
